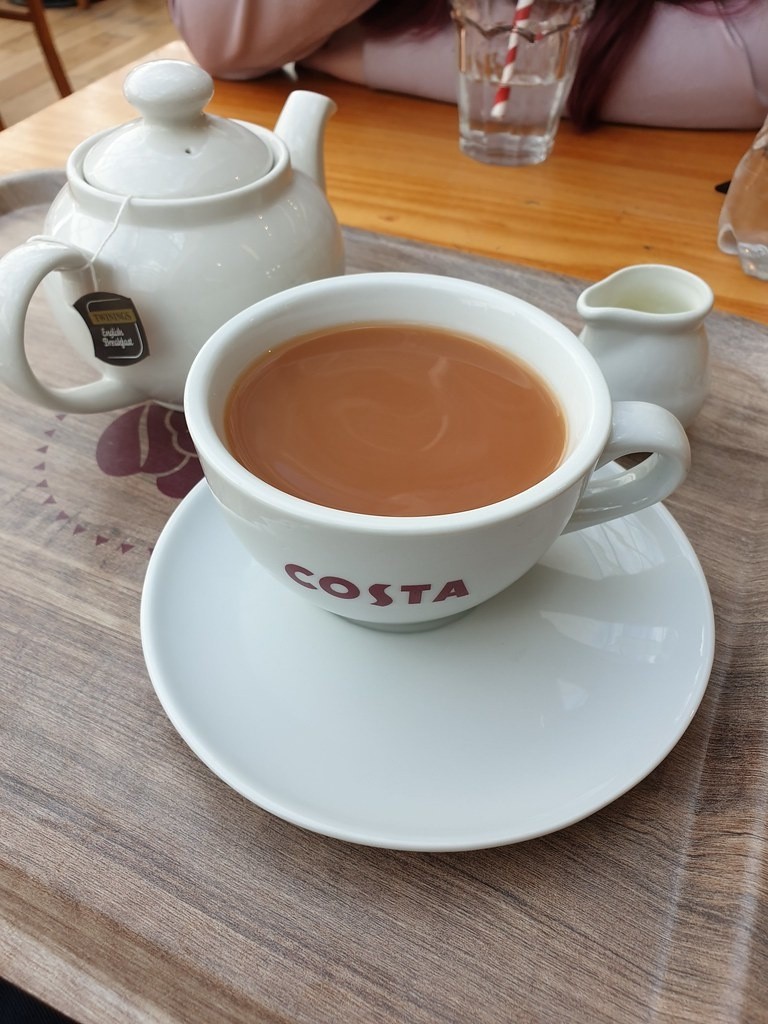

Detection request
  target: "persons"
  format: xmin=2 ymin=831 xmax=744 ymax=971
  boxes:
xmin=169 ymin=0 xmax=768 ymax=129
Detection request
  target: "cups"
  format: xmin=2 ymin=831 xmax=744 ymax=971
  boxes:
xmin=449 ymin=0 xmax=598 ymax=165
xmin=182 ymin=271 xmax=692 ymax=635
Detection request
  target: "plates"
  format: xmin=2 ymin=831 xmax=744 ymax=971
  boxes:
xmin=139 ymin=460 xmax=715 ymax=853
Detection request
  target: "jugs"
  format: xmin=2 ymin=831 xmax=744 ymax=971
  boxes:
xmin=575 ymin=262 xmax=714 ymax=430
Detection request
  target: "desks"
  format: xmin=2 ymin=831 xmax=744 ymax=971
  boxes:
xmin=0 ymin=32 xmax=768 ymax=1022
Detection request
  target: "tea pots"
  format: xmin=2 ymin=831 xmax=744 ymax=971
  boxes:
xmin=0 ymin=61 xmax=345 ymax=414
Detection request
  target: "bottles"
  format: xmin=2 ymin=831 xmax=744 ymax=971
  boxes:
xmin=715 ymin=114 xmax=768 ymax=281
